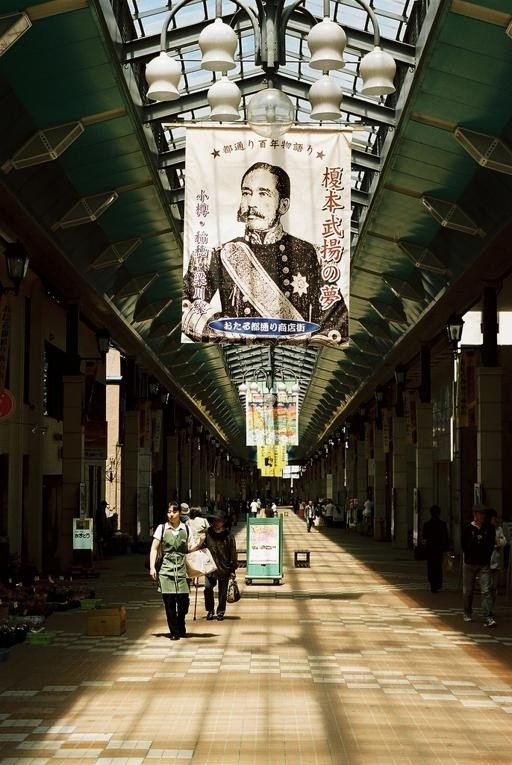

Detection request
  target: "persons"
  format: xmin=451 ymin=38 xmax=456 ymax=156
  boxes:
xmin=147 ymin=498 xmax=196 ymax=640
xmin=214 ymin=490 xmax=373 ymax=532
xmin=177 ymin=501 xmax=192 ymax=522
xmin=460 ymin=503 xmax=499 ymax=627
xmin=180 ymin=155 xmax=348 ymax=351
xmin=203 ymin=509 xmax=240 ymax=621
xmin=416 ymin=503 xmax=454 ymax=592
xmin=184 ymin=503 xmax=210 ymax=589
xmin=94 ymin=500 xmax=114 ymax=544
xmin=488 ymin=508 xmax=507 ymax=599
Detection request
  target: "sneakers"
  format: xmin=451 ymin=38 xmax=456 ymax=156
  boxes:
xmin=462 ymin=613 xmax=473 ymax=623
xmin=207 ymin=610 xmax=225 ymax=620
xmin=482 ymin=616 xmax=497 ymax=627
xmin=170 ymin=631 xmax=190 ymax=640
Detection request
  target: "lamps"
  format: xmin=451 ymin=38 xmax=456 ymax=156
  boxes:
xmin=139 ymin=0 xmax=400 ymax=138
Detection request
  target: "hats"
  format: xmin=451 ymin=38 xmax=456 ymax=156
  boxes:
xmin=180 ymin=502 xmax=191 ymax=515
xmin=206 ymin=510 xmax=230 ymax=528
xmin=471 ymin=503 xmax=488 ymax=512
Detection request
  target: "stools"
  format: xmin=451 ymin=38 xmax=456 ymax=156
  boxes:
xmin=293 ymin=548 xmax=312 ymax=571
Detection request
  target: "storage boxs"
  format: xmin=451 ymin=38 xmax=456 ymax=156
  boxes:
xmin=84 ymin=603 xmax=129 ymax=638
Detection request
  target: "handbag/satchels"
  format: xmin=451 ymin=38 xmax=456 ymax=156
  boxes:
xmin=414 ymin=544 xmax=427 ymax=561
xmin=183 ymin=542 xmax=217 ymax=579
xmin=144 ymin=523 xmax=166 ymax=573
xmin=488 ymin=545 xmax=503 ymax=571
xmin=227 ymin=577 xmax=241 ymax=603
